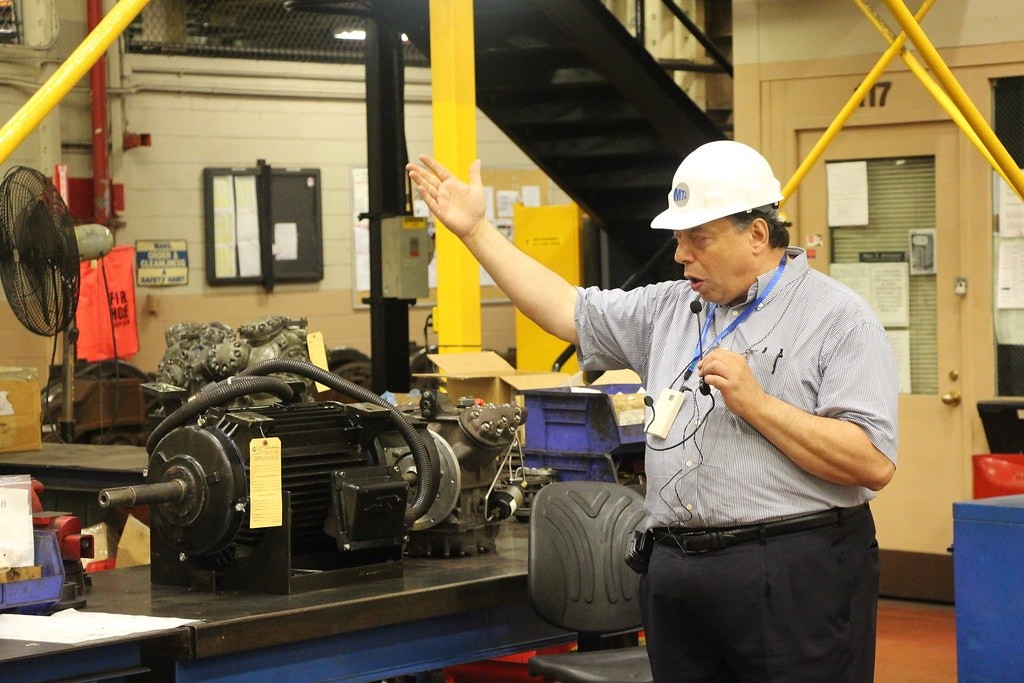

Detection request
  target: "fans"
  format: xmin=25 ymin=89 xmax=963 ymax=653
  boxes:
xmin=0 ymin=165 xmax=116 ymax=444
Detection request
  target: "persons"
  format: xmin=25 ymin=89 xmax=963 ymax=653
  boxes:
xmin=406 ymin=141 xmax=898 ymax=683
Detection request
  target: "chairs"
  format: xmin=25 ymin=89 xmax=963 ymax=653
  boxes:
xmin=527 ymin=480 xmax=655 ymax=683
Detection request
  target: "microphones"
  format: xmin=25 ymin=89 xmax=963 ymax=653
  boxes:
xmin=644 ymin=392 xmax=715 ymax=450
xmin=690 ymin=301 xmax=711 ymax=383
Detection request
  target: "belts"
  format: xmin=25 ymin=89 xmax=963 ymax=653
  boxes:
xmin=651 ymin=501 xmax=870 ymax=555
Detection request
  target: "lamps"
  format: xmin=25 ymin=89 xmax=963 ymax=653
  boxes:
xmin=327 ymin=3 xmax=367 ymax=41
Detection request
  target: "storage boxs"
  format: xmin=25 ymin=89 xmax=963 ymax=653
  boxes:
xmin=79 ymin=513 xmax=151 ymax=571
xmin=0 ymin=366 xmax=43 ymax=454
xmin=411 ymin=349 xmax=648 ymax=488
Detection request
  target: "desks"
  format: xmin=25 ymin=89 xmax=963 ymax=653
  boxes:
xmin=0 ymin=442 xmax=152 ymax=531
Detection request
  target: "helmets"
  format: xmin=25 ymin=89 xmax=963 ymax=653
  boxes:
xmin=650 ymin=140 xmax=785 ymax=229
